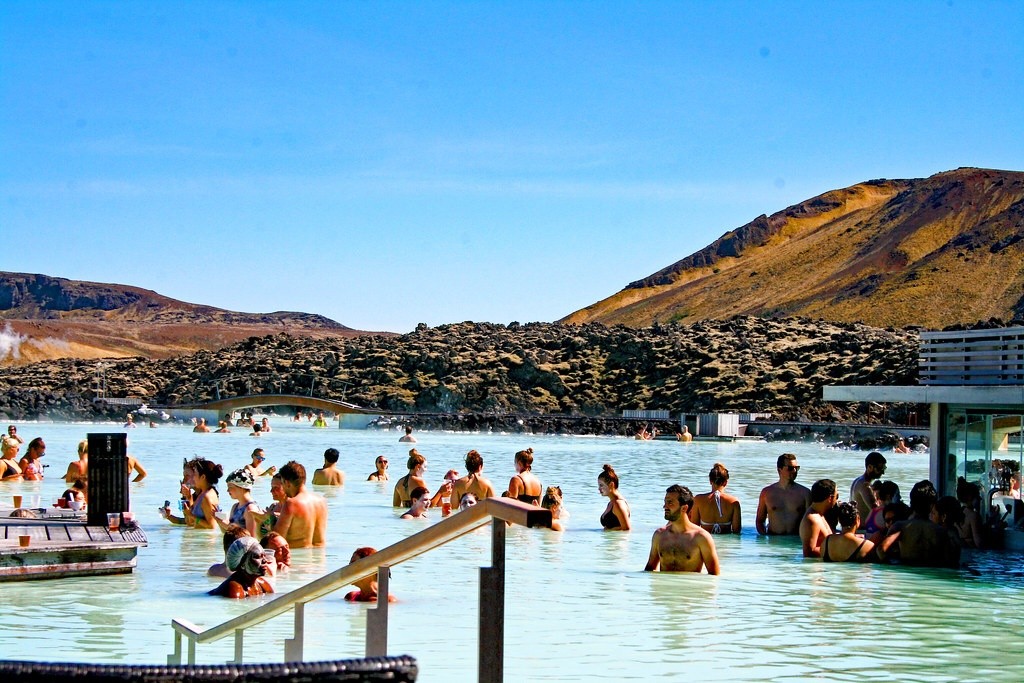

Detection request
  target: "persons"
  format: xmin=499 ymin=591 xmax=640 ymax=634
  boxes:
xmin=157 ymin=448 xmax=400 ymax=604
xmin=450 ymin=450 xmax=494 ymax=510
xmin=501 ymin=486 xmax=570 ymax=532
xmin=509 ymin=447 xmax=542 ymax=504
xmin=824 ymin=480 xmax=966 ymax=569
xmin=400 ymin=487 xmax=431 ymax=519
xmin=644 ymin=484 xmax=720 ymax=576
xmin=676 ymin=425 xmax=692 ymax=442
xmin=956 ymin=476 xmax=986 ymax=551
xmin=635 ymin=421 xmax=657 ymax=441
xmin=0 ymin=425 xmax=147 ymax=509
xmin=850 ymin=451 xmax=887 ymax=532
xmin=895 ymin=439 xmax=908 ymax=454
xmin=437 ymin=471 xmax=479 ymax=512
xmin=367 ymin=455 xmax=389 ymax=482
xmin=392 ymin=449 xmax=451 ymax=508
xmin=798 ymin=479 xmax=838 ymax=558
xmin=124 ymin=410 xmax=339 ymax=436
xmin=598 ymin=464 xmax=631 ymax=531
xmin=757 ymin=454 xmax=812 ymax=536
xmin=312 ymin=448 xmax=344 ymax=486
xmin=689 ymin=463 xmax=742 ymax=535
xmin=398 ymin=426 xmax=416 ymax=443
xmin=1007 ymin=471 xmax=1022 ymax=497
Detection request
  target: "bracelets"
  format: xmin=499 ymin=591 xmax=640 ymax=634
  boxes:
xmin=195 ymin=517 xmax=200 ymax=526
xmin=178 ymin=518 xmax=183 ymax=524
xmin=266 ymin=470 xmax=270 ymax=474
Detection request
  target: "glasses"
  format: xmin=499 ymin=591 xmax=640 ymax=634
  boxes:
xmin=377 ymin=461 xmax=388 ymax=464
xmin=784 ymin=465 xmax=800 ymax=472
xmin=36 ymin=451 xmax=45 ymax=456
xmin=253 ymin=453 xmax=266 ymax=461
xmin=830 ymin=493 xmax=839 ymax=500
xmin=195 ymin=458 xmax=203 ymax=469
xmin=8 ymin=429 xmax=15 ymax=431
xmin=878 ymin=466 xmax=887 ymax=471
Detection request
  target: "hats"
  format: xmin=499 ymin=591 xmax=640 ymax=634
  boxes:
xmin=225 ymin=469 xmax=255 ymax=489
xmin=127 ymin=414 xmax=132 ymax=419
xmin=199 ymin=418 xmax=205 ymax=421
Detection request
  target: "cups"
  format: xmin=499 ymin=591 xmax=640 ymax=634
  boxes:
xmin=107 ymin=513 xmax=120 ymax=533
xmin=263 ymin=548 xmax=275 ymax=576
xmin=30 ymin=495 xmax=41 ymax=508
xmin=441 ymin=497 xmax=451 ymax=515
xmin=58 ymin=499 xmax=66 ymax=508
xmin=13 ymin=494 xmax=23 ymax=509
xmin=122 ymin=511 xmax=134 ymax=524
xmin=18 ymin=534 xmax=31 ymax=549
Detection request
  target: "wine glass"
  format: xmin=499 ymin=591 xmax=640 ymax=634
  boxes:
xmin=68 ymin=501 xmax=83 ymax=519
